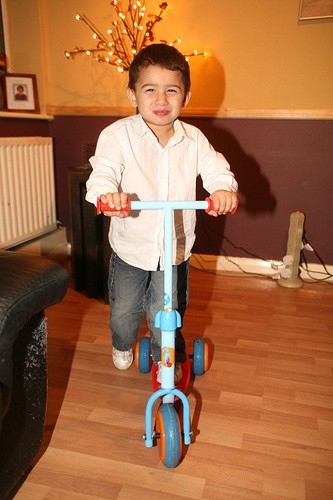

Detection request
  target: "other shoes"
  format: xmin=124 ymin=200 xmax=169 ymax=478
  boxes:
xmin=111 ymin=345 xmax=134 ymax=370
xmin=156 ymin=360 xmax=183 ymax=385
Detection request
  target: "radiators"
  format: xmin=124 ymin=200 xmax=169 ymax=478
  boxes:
xmin=0 ymin=137 xmax=58 ymax=250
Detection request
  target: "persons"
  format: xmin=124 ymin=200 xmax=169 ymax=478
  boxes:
xmin=84 ymin=43 xmax=238 ymax=386
xmin=15 ymin=86 xmax=27 ymax=99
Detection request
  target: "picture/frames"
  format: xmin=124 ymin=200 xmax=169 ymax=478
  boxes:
xmin=1 ymin=73 xmax=40 ymax=114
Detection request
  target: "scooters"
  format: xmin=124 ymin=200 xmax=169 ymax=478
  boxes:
xmin=95 ymin=195 xmax=240 ymax=470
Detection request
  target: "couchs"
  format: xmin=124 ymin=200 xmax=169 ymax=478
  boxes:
xmin=0 ymin=251 xmax=71 ymax=500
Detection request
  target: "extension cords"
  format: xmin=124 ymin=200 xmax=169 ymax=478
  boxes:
xmin=277 ymin=211 xmax=305 ymax=288
